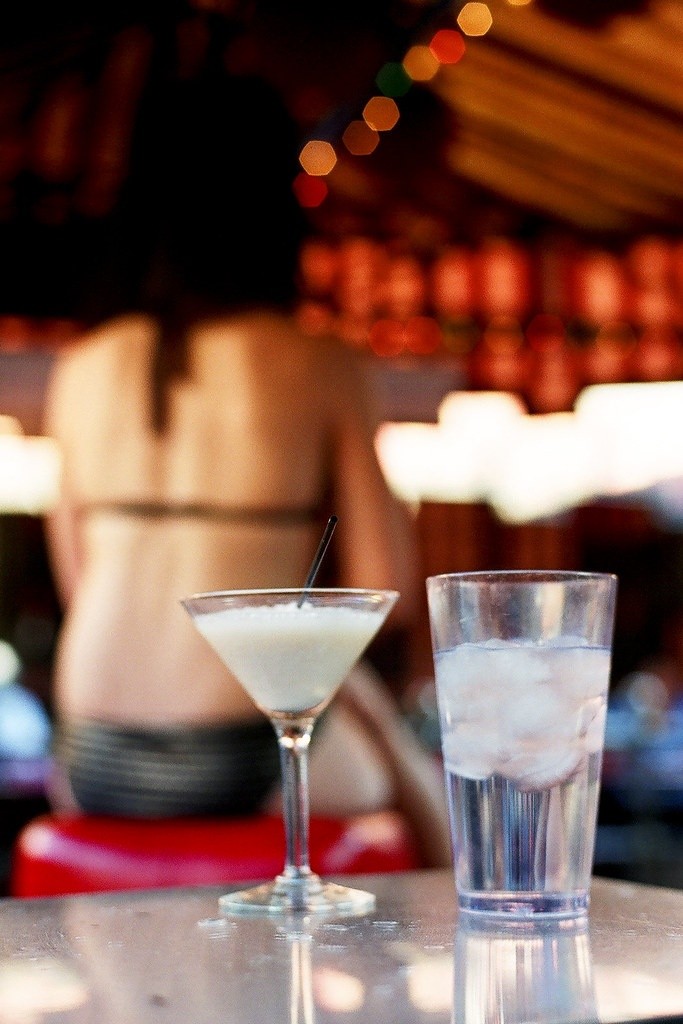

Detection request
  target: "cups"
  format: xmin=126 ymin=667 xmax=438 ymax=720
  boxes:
xmin=427 ymin=569 xmax=617 ymax=932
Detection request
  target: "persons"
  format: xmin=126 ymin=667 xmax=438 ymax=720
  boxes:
xmin=43 ymin=69 xmax=466 ymax=886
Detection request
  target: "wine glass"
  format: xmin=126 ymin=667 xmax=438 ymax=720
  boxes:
xmin=179 ymin=588 xmax=401 ymax=917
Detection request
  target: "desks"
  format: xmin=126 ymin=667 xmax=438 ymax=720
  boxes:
xmin=0 ymin=868 xmax=683 ymax=1024
